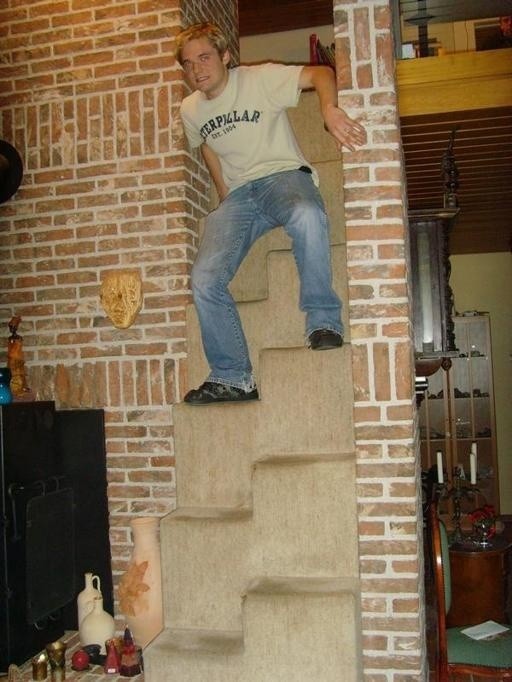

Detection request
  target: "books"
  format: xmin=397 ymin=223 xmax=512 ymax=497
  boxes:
xmin=307 ymin=31 xmax=336 ymax=67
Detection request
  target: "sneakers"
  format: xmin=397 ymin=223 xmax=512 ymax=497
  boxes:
xmin=308 ymin=327 xmax=344 ymax=352
xmin=185 ymin=382 xmax=260 ymax=406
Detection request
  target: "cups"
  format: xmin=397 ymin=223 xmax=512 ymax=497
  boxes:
xmin=45 ymin=640 xmax=69 ymax=670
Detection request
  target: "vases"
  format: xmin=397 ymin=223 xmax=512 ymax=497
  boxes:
xmin=478 ymin=523 xmax=495 ymax=548
xmin=77 ymin=518 xmax=163 ymax=657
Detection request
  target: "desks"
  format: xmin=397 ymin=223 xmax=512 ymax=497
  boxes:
xmin=445 ymin=534 xmax=512 ymax=629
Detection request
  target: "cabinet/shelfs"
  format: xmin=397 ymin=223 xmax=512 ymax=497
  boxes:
xmin=416 ymin=314 xmax=501 ymax=523
xmin=406 ymin=206 xmax=460 ymax=378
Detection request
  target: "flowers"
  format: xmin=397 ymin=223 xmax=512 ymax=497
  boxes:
xmin=468 ymin=504 xmax=499 ymax=527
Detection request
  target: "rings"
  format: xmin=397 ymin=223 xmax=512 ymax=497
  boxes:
xmin=344 ymin=127 xmax=352 ymax=132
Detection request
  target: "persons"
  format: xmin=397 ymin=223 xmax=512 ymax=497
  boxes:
xmin=477 ymin=15 xmax=512 ymax=53
xmin=175 ymin=17 xmax=370 ymax=409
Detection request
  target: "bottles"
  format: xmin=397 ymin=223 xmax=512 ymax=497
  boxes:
xmin=75 ymin=570 xmax=119 ymax=659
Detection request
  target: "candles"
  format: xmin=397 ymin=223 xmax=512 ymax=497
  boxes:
xmin=469 ymin=441 xmax=478 ymax=486
xmin=436 ymin=451 xmax=444 ymax=484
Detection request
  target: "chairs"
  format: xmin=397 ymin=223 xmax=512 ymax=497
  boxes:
xmin=428 ymin=501 xmax=512 ymax=682
